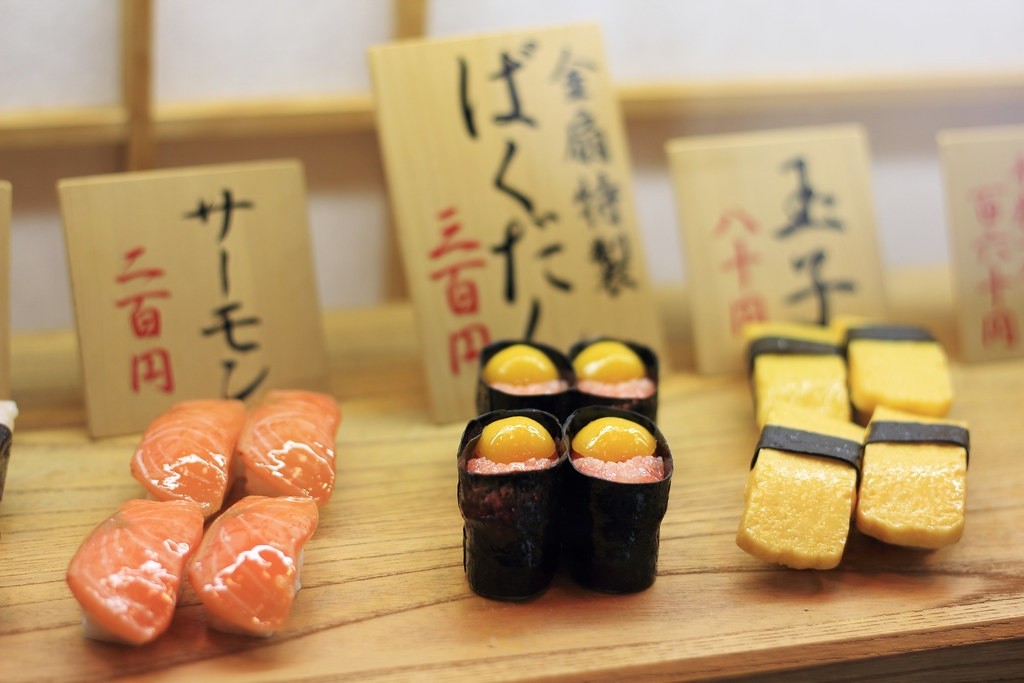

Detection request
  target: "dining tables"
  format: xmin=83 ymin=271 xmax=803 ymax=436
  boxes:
xmin=1 ymin=346 xmax=1024 ymax=683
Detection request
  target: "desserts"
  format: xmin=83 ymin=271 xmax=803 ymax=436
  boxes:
xmin=735 ymin=315 xmax=971 ymax=572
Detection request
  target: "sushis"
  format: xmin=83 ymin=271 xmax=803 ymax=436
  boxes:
xmin=456 ymin=335 xmax=675 ymax=603
